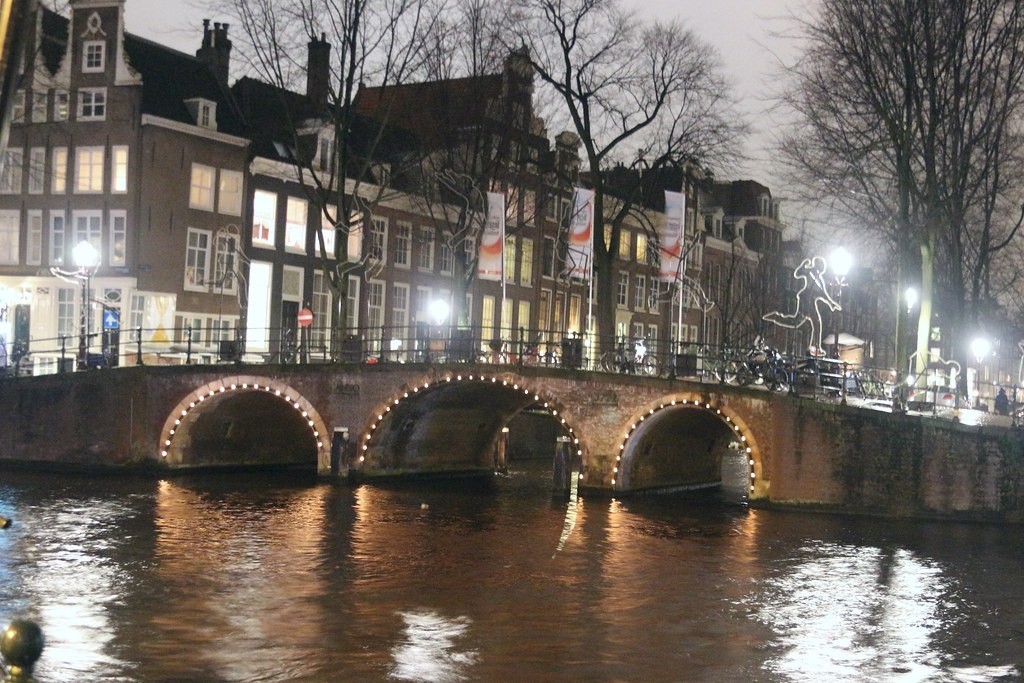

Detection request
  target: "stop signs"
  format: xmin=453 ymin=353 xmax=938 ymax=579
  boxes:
xmin=298 ymin=308 xmax=314 ymax=327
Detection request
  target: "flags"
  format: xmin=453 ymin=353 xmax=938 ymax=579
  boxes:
xmin=477 ymin=192 xmax=504 ymax=280
xmin=565 ymin=188 xmax=594 ymax=278
xmin=661 ymin=189 xmax=683 ymax=283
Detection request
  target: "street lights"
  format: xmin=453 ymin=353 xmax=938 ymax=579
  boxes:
xmin=827 ymin=247 xmax=850 ymax=394
xmin=973 ymin=337 xmax=987 ymax=409
xmin=74 ymin=239 xmax=96 ymax=371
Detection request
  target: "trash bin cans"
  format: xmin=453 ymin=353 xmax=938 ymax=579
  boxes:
xmin=449 ymin=330 xmax=473 ymax=362
xmin=339 ymin=335 xmax=363 ymax=363
xmin=676 ymin=353 xmax=697 ymax=376
xmin=793 ymin=367 xmax=818 ymax=400
xmin=562 ymin=338 xmax=582 ymax=368
xmin=218 ymin=340 xmax=242 ymax=361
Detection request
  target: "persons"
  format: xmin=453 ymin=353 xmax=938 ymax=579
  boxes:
xmin=994 ymin=388 xmax=1008 ymax=415
xmin=885 ymin=371 xmax=896 ymax=397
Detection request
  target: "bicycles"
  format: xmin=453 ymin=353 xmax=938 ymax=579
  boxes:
xmin=715 ymin=338 xmax=790 ymax=393
xmin=435 ymin=339 xmax=588 ymax=370
xmin=602 ymin=336 xmax=664 ymax=377
